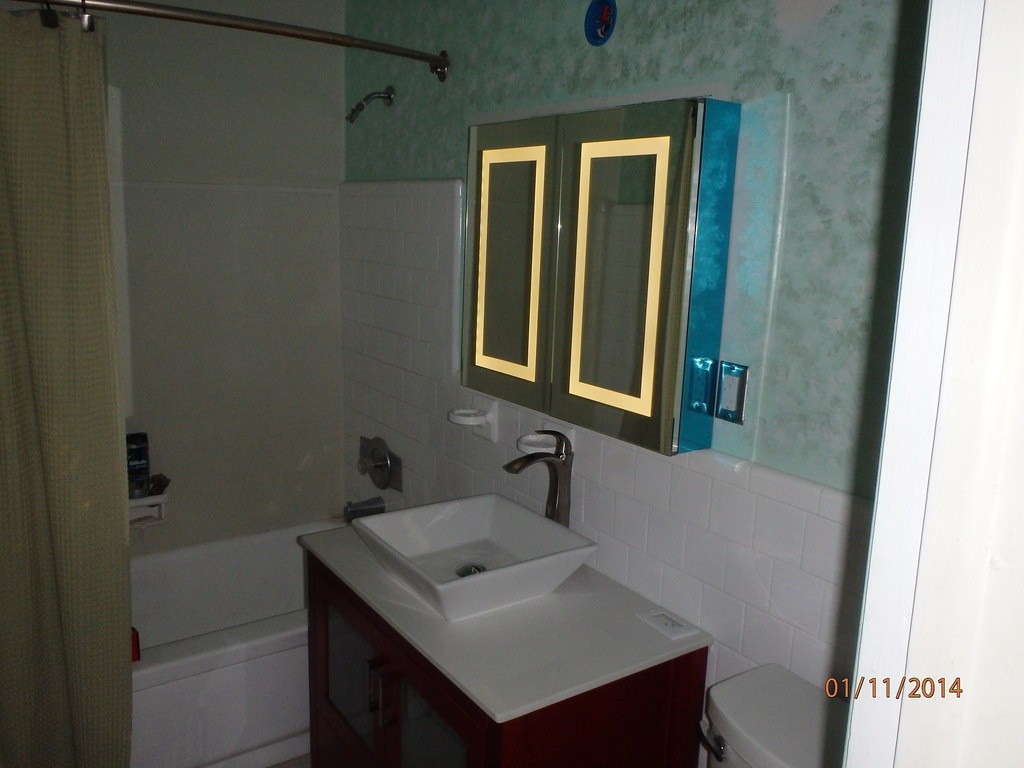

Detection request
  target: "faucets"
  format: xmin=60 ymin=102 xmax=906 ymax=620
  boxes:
xmin=501 ymin=429 xmax=575 ymax=528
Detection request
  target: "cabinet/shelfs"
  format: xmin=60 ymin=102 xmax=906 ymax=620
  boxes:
xmin=461 ymin=97 xmax=743 ymax=458
xmin=305 ymin=550 xmax=710 ymax=768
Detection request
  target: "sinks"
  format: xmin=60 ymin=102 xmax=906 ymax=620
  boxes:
xmin=351 ymin=492 xmax=601 ymax=623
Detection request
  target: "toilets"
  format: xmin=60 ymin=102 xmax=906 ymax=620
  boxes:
xmin=709 ymin=662 xmax=830 ymax=768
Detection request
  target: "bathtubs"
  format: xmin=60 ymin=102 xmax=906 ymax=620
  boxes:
xmin=129 ymin=517 xmax=348 ymax=768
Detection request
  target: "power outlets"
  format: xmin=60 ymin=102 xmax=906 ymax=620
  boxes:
xmin=715 ymin=362 xmax=748 ymax=425
